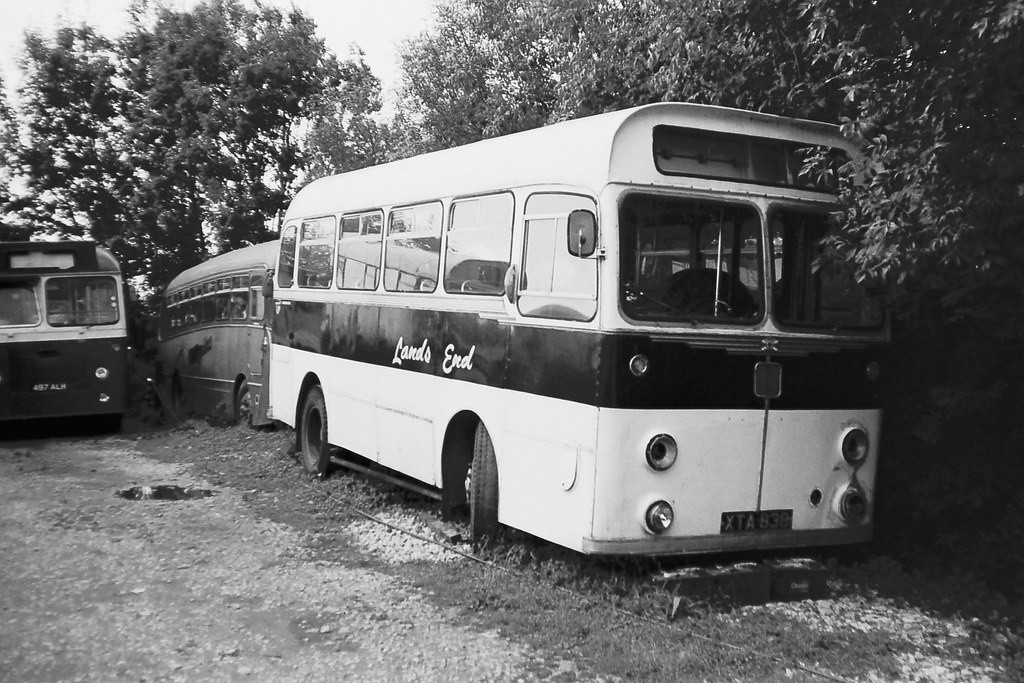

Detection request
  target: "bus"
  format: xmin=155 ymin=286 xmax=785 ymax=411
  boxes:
xmin=0 ymin=238 xmax=129 ymax=433
xmin=148 ymin=239 xmax=276 ymax=429
xmin=268 ymin=101 xmax=897 ymax=558
